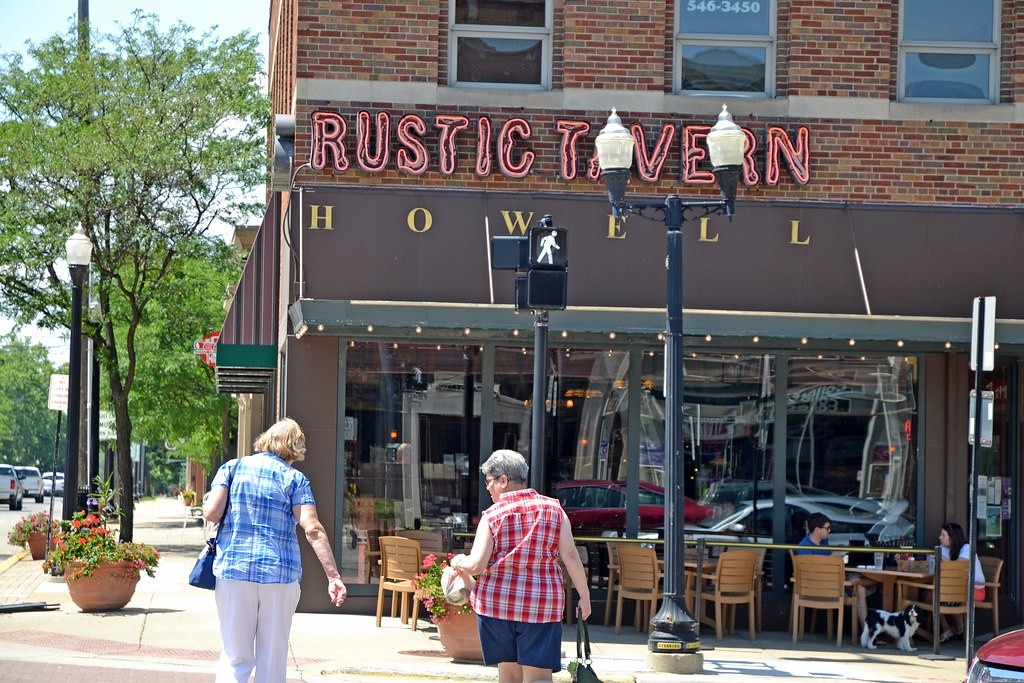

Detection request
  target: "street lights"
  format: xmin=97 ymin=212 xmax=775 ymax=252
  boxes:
xmin=86 ymin=301 xmax=102 ymax=526
xmin=65 ymin=223 xmax=95 ymax=537
xmin=595 ymin=106 xmax=748 ymax=674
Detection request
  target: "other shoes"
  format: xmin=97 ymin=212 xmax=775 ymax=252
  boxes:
xmin=939 ymin=630 xmax=954 ymax=645
xmin=952 ymin=627 xmax=964 ymax=638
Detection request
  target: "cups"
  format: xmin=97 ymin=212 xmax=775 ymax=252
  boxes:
xmin=874 ymin=552 xmax=884 ymax=570
xmin=929 ymin=555 xmax=935 ymax=574
xmin=842 ymin=555 xmax=848 ymax=563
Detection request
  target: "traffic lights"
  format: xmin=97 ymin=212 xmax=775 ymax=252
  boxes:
xmin=489 ymin=234 xmax=528 ymax=313
xmin=527 ymin=226 xmax=569 ymax=311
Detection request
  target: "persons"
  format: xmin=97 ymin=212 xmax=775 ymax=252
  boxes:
xmin=203 ymin=419 xmax=347 ymax=683
xmin=796 ymin=512 xmax=879 ymax=629
xmin=452 ymin=448 xmax=592 ymax=683
xmin=933 ymin=523 xmax=986 ymax=643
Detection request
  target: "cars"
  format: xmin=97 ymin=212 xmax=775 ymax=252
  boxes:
xmin=474 ymin=479 xmax=715 ymax=538
xmin=602 ymin=476 xmax=915 ymax=547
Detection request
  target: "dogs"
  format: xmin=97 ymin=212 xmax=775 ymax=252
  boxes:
xmin=859 ymin=604 xmax=921 ymax=652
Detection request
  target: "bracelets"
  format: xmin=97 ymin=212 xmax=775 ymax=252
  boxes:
xmin=455 ymin=556 xmax=466 ymax=567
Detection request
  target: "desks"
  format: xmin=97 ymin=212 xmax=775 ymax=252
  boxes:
xmin=378 ymin=549 xmax=468 ymax=624
xmin=655 ymin=557 xmax=719 ymax=616
xmin=844 ymin=565 xmax=934 ymax=643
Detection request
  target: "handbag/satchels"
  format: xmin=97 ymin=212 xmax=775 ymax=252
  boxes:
xmin=188 ymin=540 xmax=216 ymax=590
xmin=440 ymin=562 xmax=477 ymax=607
xmin=572 ymin=608 xmax=601 ymax=683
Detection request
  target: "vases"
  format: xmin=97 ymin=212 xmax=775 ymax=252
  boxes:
xmin=61 ymin=555 xmax=141 ymax=612
xmin=436 ymin=606 xmax=484 ymax=665
xmin=27 ymin=531 xmax=46 ymax=560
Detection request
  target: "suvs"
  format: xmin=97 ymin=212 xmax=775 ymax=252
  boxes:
xmin=0 ymin=464 xmax=45 ymax=510
xmin=42 ymin=472 xmax=64 ymax=497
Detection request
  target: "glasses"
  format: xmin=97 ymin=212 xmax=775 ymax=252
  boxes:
xmin=820 ymin=526 xmax=830 ymax=531
xmin=486 ymin=473 xmax=502 ymax=486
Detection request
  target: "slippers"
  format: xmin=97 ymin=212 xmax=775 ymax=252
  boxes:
xmin=860 ymin=636 xmax=886 ymax=646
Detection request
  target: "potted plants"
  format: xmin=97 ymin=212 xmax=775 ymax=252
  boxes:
xmin=182 ymin=488 xmax=194 ymax=506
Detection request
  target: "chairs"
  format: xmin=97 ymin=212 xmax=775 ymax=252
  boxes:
xmin=375 ymin=525 xmax=1005 ymax=644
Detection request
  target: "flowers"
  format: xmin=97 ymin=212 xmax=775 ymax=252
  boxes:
xmin=6 ymin=510 xmax=62 ymax=550
xmin=41 ymin=511 xmax=160 ymax=579
xmin=407 ymin=552 xmax=484 ymax=616
